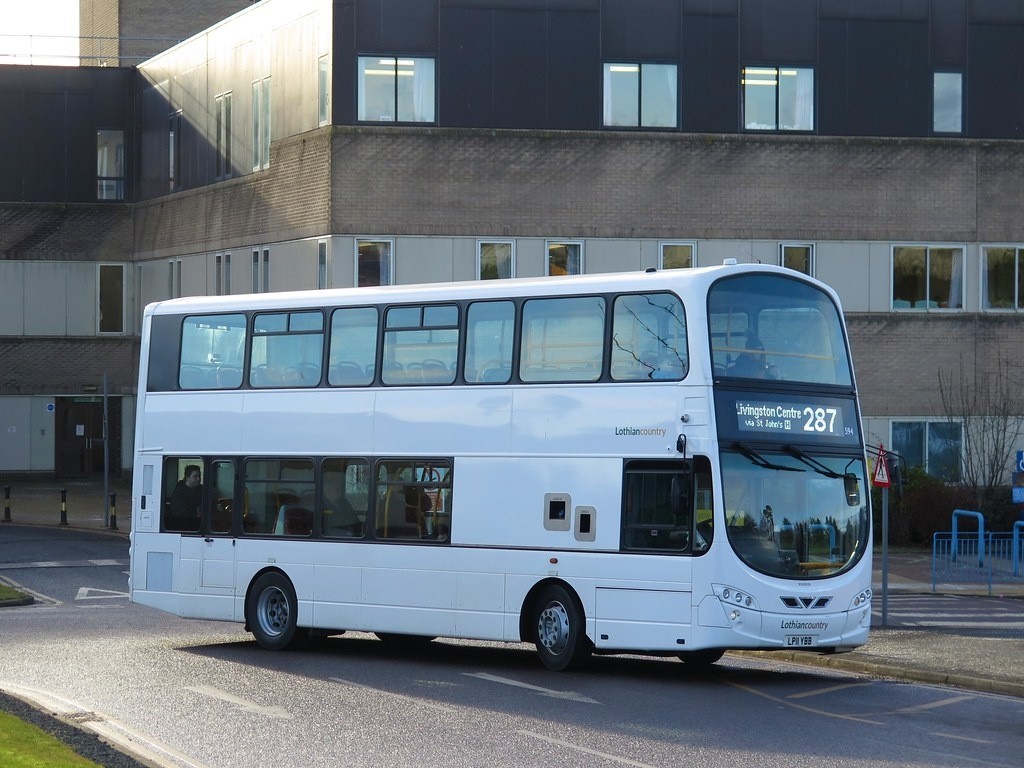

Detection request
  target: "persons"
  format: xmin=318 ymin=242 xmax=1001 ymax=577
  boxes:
xmin=170 ymin=465 xmax=203 ymax=523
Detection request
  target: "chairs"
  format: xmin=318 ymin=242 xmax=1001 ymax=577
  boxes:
xmin=178 ymin=358 xmax=602 ymax=383
xmin=161 ymin=488 xmax=428 ymax=541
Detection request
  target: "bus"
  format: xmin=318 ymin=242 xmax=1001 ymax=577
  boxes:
xmin=126 ymin=256 xmax=910 ymax=675
